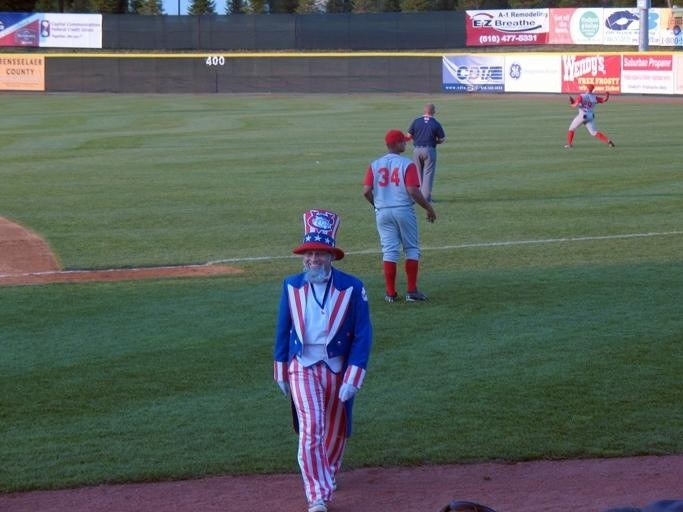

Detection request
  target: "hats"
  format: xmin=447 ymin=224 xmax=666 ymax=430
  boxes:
xmin=583 ymin=84 xmax=595 ymax=90
xmin=383 ymin=128 xmax=412 ymax=146
xmin=290 ymin=208 xmax=345 ymax=262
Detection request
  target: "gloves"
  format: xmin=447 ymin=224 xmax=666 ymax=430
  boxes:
xmin=336 ymin=382 xmax=357 ymax=402
xmin=275 ymin=380 xmax=288 ymax=398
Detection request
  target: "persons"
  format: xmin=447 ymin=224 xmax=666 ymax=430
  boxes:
xmin=407 ymin=102 xmax=445 ymax=203
xmin=364 ymin=130 xmax=436 ymax=302
xmin=563 ymin=83 xmax=615 ymax=148
xmin=274 ymin=249 xmax=373 ymax=512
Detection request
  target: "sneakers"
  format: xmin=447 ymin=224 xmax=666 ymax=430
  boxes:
xmin=308 ymin=500 xmax=326 ymax=512
xmin=383 ymin=295 xmax=394 ymax=303
xmin=564 ymin=145 xmax=573 ymax=150
xmin=607 ymin=140 xmax=616 ymax=149
xmin=405 ymin=291 xmax=429 ymax=302
